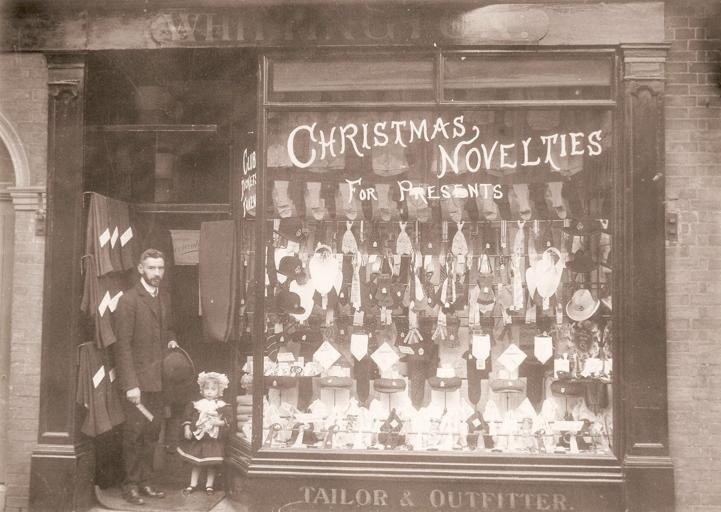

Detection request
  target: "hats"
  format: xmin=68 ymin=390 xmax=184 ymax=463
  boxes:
xmin=429 ymin=376 xmax=461 ymax=392
xmin=567 ymin=289 xmax=601 ymax=322
xmin=562 ymin=220 xmax=602 ymax=238
xmin=274 ymin=216 xmax=307 ymax=243
xmin=277 ymin=291 xmax=306 ymax=314
xmin=373 ymin=379 xmax=406 ymax=394
xmin=266 ymin=376 xmax=297 ymax=388
xmin=277 ymin=256 xmax=308 ymax=285
xmin=161 ymin=347 xmax=196 ymax=390
xmin=492 ymin=378 xmax=527 ymax=394
xmin=319 ymin=376 xmax=356 ymax=388
xmin=566 ymin=249 xmax=604 ymax=274
xmin=550 ymin=380 xmax=586 ymax=395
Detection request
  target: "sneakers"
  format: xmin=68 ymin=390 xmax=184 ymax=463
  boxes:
xmin=183 ymin=485 xmax=198 ymax=496
xmin=205 ymin=487 xmax=216 ymax=495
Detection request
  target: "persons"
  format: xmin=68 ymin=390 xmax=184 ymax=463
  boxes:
xmin=113 ymin=247 xmax=180 ymax=505
xmin=176 ymin=370 xmax=234 ymax=495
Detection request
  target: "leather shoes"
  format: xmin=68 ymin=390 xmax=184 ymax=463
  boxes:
xmin=123 ymin=488 xmax=145 ymax=505
xmin=139 ymin=485 xmax=166 ymax=499
